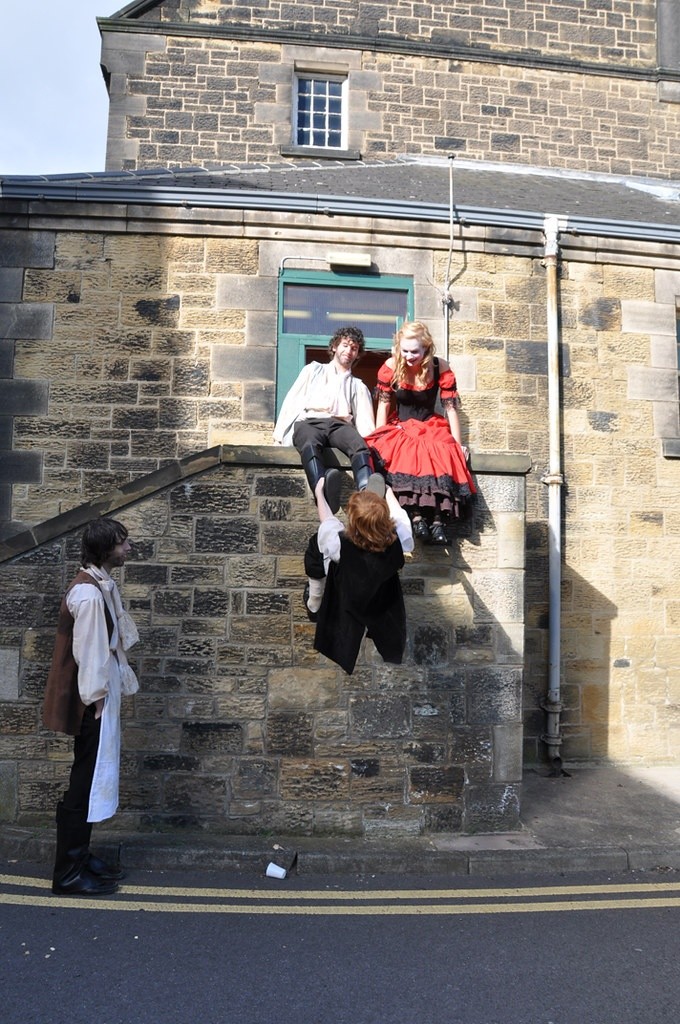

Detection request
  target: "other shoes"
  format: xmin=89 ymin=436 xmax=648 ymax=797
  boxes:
xmin=431 ymin=523 xmax=448 ymax=546
xmin=303 ymin=581 xmax=322 ymax=622
xmin=413 ymin=518 xmax=429 ymax=539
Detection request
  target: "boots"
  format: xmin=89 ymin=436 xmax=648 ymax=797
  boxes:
xmin=299 ymin=444 xmax=343 ymax=516
xmin=80 ymin=851 xmax=126 ymax=881
xmin=351 ymin=453 xmax=386 ymax=500
xmin=50 ymin=800 xmax=118 ymax=896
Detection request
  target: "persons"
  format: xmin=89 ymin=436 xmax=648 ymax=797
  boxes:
xmin=271 ymin=326 xmax=386 ymax=514
xmin=303 ymin=475 xmax=414 ymax=676
xmin=42 ymin=517 xmax=141 ymax=897
xmin=363 ymin=322 xmax=477 ymax=544
